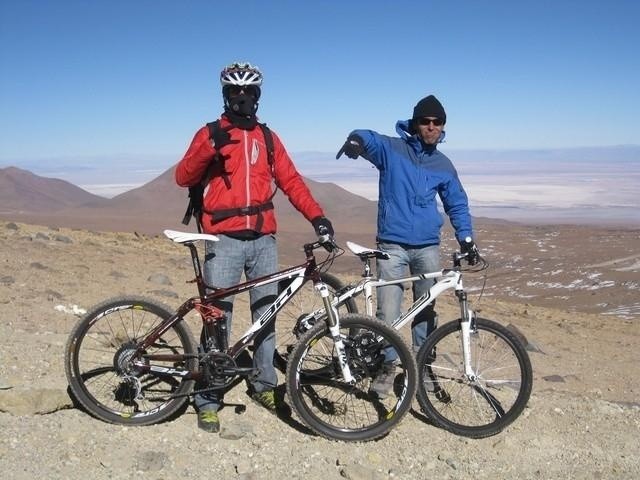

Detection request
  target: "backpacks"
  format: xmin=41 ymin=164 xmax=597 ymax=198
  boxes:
xmin=183 ymin=120 xmax=278 ymax=232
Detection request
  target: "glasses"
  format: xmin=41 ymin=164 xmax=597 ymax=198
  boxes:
xmin=414 ymin=117 xmax=443 ymax=125
xmin=227 ymin=83 xmax=258 ymax=98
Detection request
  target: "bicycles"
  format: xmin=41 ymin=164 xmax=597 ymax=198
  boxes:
xmin=63 ymin=227 xmax=424 ymax=446
xmin=268 ymin=234 xmax=536 ymax=441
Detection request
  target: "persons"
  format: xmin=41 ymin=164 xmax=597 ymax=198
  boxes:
xmin=171 ymin=62 xmax=335 ymax=432
xmin=337 ymin=96 xmax=478 ymax=404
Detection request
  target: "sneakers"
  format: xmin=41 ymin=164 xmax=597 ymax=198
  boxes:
xmin=419 ymin=359 xmax=443 ymax=394
xmin=365 ymin=362 xmax=398 ymax=401
xmin=195 ymin=406 xmax=221 ymax=435
xmin=248 ymin=386 xmax=293 ymax=419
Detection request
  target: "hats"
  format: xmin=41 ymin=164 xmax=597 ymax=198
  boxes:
xmin=411 ymin=94 xmax=448 ymax=126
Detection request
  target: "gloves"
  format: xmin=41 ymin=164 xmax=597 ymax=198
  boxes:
xmin=460 ymin=237 xmax=480 ymax=266
xmin=209 ymin=121 xmax=243 ymax=151
xmin=310 ymin=216 xmax=340 ymax=253
xmin=334 ymin=131 xmax=367 ymax=163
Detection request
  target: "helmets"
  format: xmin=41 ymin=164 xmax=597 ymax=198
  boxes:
xmin=218 ymin=61 xmax=264 ymax=88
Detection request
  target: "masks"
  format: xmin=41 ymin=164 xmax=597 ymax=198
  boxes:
xmin=228 ymin=95 xmax=256 ymax=116
